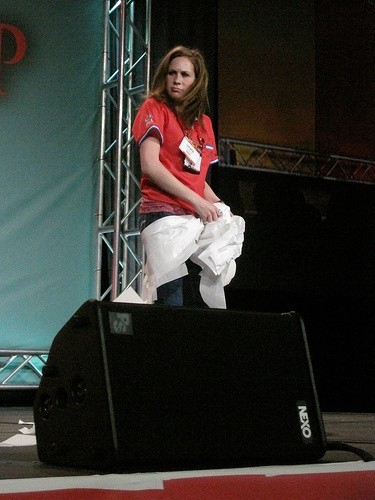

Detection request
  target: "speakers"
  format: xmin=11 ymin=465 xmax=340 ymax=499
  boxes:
xmin=30 ymin=299 xmax=328 ymax=471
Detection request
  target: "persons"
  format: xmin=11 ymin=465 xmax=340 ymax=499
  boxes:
xmin=131 ymin=46 xmax=226 ymax=309
